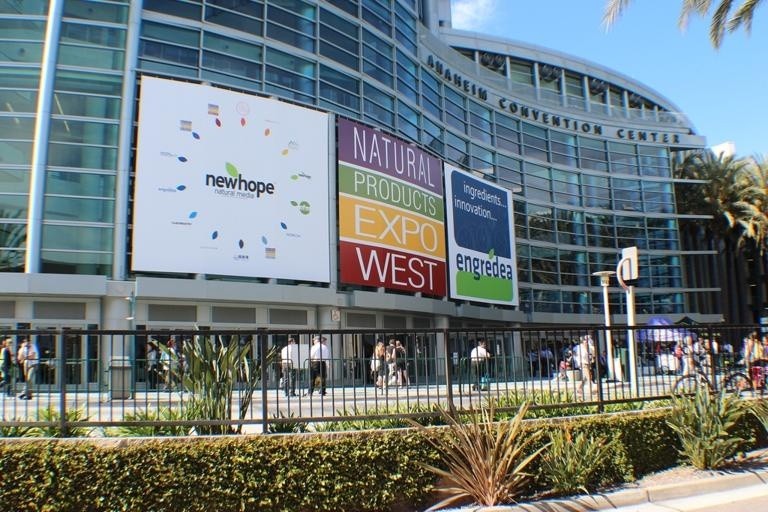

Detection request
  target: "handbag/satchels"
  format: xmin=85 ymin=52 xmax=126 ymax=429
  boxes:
xmin=378 ymin=365 xmax=389 ymax=375
xmin=481 ymin=377 xmax=490 ymax=391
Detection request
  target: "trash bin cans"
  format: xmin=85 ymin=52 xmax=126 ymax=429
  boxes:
xmin=107 ymin=356 xmax=131 ymax=399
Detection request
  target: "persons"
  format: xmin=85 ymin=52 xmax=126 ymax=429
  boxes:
xmin=145 ymin=339 xmax=158 ymax=391
xmin=0 ymin=337 xmax=39 ymax=399
xmin=159 ymin=339 xmax=178 ymax=392
xmin=470 ymin=339 xmax=490 ymax=391
xmin=281 ymin=336 xmax=330 ymax=397
xmin=371 ymin=337 xmax=412 ymax=390
xmin=674 ymin=330 xmax=768 ymax=387
xmin=528 ymin=334 xmax=607 ymax=396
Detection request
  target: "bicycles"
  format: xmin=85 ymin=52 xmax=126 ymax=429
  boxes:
xmin=673 ymin=361 xmax=755 ymax=404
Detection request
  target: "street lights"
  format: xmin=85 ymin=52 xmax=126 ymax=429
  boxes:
xmin=592 ymin=270 xmax=617 ymax=382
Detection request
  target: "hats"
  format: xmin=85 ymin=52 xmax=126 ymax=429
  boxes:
xmin=312 ymin=336 xmax=320 ymax=340
